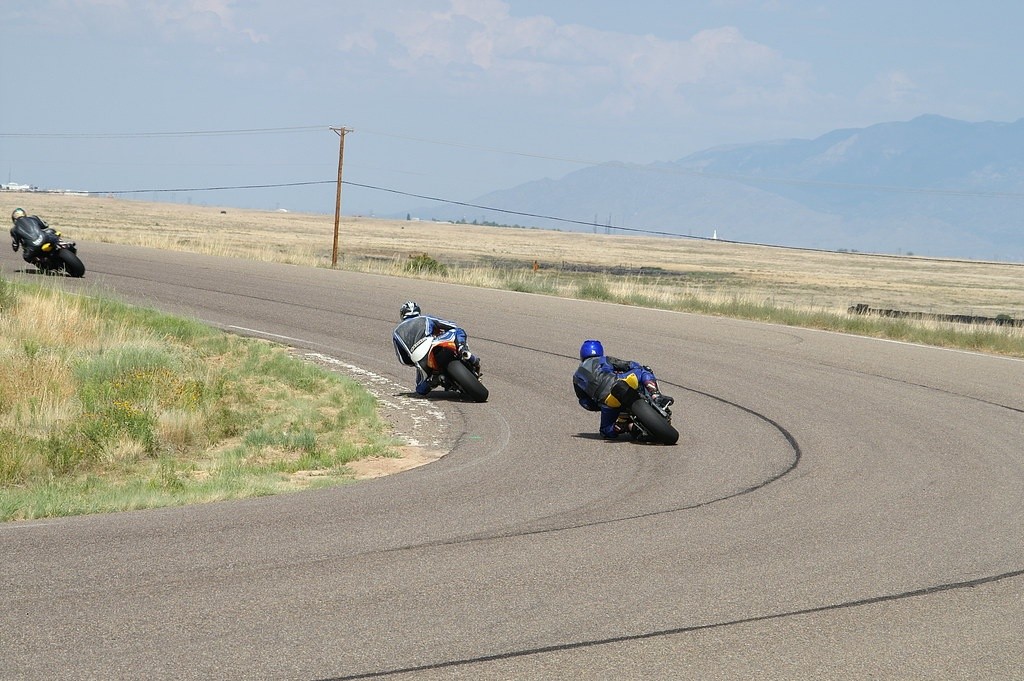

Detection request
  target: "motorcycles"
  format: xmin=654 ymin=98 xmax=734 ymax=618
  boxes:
xmin=12 ymin=230 xmax=85 ymax=278
xmin=424 ymin=327 xmax=490 ymax=404
xmin=603 ymin=366 xmax=680 ymax=446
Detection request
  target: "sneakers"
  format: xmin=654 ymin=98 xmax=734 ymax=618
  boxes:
xmin=426 ymin=374 xmax=440 ymax=389
xmin=456 ymin=342 xmax=471 ymax=353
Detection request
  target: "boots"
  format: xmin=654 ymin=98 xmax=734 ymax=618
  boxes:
xmin=644 ymin=380 xmax=674 ymax=406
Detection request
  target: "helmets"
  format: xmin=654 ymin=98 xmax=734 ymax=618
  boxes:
xmin=399 ymin=300 xmax=421 ymax=321
xmin=12 ymin=208 xmax=26 ymax=225
xmin=579 ymin=339 xmax=603 ymax=362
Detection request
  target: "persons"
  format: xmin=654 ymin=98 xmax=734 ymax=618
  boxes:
xmin=571 ymin=339 xmax=659 ymax=442
xmin=10 ymin=208 xmax=61 ymax=274
xmin=392 ymin=302 xmax=479 ymax=395
